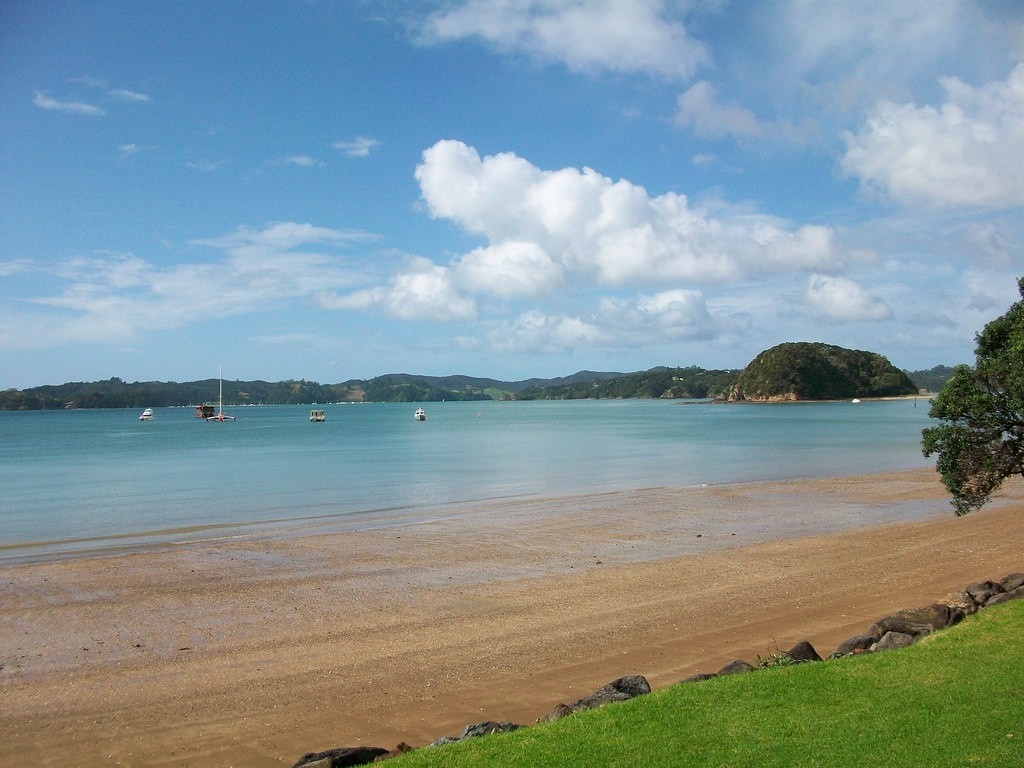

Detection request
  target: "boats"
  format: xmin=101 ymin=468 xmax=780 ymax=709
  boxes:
xmin=138 ymin=407 xmax=156 ymax=421
xmin=414 ymin=406 xmax=426 ymax=421
xmin=309 ymin=409 xmax=328 ymax=422
xmin=195 ymin=403 xmax=218 ymax=419
